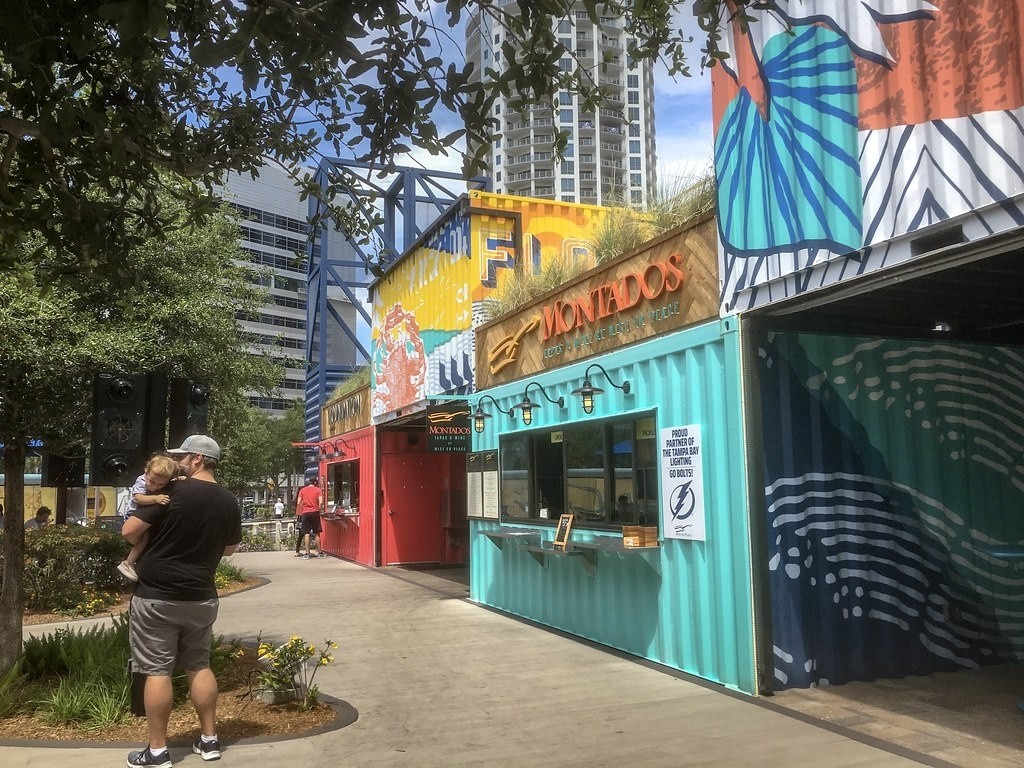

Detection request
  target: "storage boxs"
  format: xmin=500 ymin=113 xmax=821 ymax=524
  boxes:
xmin=621 ymin=525 xmax=657 ymax=548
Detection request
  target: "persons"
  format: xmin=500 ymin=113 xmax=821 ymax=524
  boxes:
xmin=274 ymin=498 xmax=285 ymax=519
xmin=294 ymin=476 xmax=327 ymax=560
xmin=25 ymin=507 xmax=51 ymax=528
xmin=116 ymin=435 xmax=243 ymax=768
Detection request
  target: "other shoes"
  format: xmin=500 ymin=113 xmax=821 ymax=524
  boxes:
xmin=295 ymin=553 xmax=303 ymax=557
xmin=308 ymin=552 xmax=316 ymax=557
xmin=303 ymin=554 xmax=310 ymax=559
xmin=318 ymin=553 xmax=323 ymax=558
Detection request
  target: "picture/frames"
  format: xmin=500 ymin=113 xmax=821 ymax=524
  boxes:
xmin=554 ymin=514 xmax=575 ymax=545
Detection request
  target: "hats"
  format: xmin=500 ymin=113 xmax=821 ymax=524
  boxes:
xmin=310 ymin=476 xmax=318 ymax=483
xmin=167 ymin=433 xmax=220 ymax=462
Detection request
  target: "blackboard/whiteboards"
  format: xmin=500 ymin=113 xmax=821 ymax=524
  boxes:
xmin=552 ymin=514 xmax=573 ymax=546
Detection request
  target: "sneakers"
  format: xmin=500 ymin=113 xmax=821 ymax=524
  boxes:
xmin=192 ymin=736 xmax=220 ymax=760
xmin=126 ymin=743 xmax=172 ymax=768
xmin=117 ymin=561 xmax=138 ymax=582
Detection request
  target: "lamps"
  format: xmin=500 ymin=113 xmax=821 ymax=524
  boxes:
xmin=512 ymin=381 xmax=564 ymax=425
xmin=333 ymin=438 xmax=355 ymax=458
xmin=322 ymin=442 xmax=342 ymax=459
xmin=310 ymin=445 xmax=326 ymax=463
xmin=469 ymin=396 xmax=514 ymax=433
xmin=571 ymin=363 xmax=629 ymax=414
